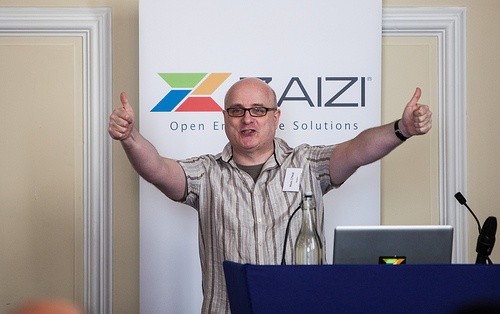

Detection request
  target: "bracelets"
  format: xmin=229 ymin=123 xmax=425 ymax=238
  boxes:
xmin=394 ymin=119 xmax=410 ymax=142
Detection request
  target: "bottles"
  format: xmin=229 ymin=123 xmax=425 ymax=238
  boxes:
xmin=294 ymin=191 xmax=322 ymax=265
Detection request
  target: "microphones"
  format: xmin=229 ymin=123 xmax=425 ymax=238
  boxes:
xmin=476 ymin=216 xmax=497 ymax=265
xmin=281 ymin=195 xmax=312 ymax=266
xmin=454 ymin=192 xmax=493 ymax=264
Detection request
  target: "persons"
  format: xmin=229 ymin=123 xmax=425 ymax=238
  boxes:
xmin=107 ymin=76 xmax=432 ymax=314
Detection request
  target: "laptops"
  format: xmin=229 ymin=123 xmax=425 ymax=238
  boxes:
xmin=332 ymin=224 xmax=453 ymax=265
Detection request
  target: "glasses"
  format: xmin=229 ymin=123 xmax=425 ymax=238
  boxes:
xmin=223 ymin=105 xmax=276 ymax=118
